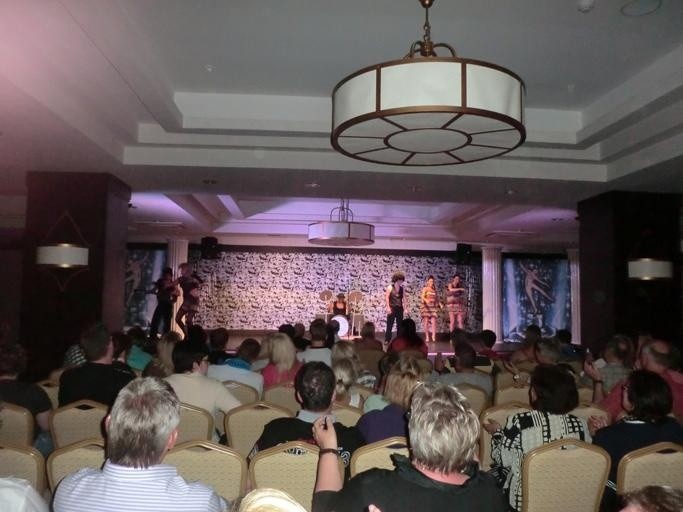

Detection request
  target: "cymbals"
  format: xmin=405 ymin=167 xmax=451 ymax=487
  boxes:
xmin=320 ymin=290 xmax=332 ymax=301
xmin=350 ymin=291 xmax=362 ymax=301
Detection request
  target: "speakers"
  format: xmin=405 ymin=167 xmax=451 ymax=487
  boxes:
xmin=456 ymin=243 xmax=472 ymax=257
xmin=200 ymin=237 xmax=217 ymax=259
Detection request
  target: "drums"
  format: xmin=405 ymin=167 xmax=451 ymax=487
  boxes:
xmin=332 ymin=315 xmax=349 ymax=336
xmin=316 ymin=313 xmax=332 ymax=324
xmin=350 ymin=313 xmax=363 ymax=327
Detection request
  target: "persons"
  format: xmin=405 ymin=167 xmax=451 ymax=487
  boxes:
xmin=144 ymin=267 xmax=177 ymax=337
xmin=163 ymin=262 xmax=200 ymax=337
xmin=0 ymin=317 xmax=683 ymax=512
xmin=519 ymin=260 xmax=556 ymax=315
xmin=328 ymin=293 xmax=349 ymax=315
xmin=446 ymin=274 xmax=466 ymax=344
xmin=384 ymin=272 xmax=409 ymax=345
xmin=420 ymin=275 xmax=444 ymax=342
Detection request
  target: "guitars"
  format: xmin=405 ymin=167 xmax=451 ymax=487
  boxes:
xmin=133 ymin=288 xmax=177 ymax=304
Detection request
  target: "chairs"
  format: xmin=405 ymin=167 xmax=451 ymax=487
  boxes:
xmin=1 ymin=350 xmax=682 ymax=512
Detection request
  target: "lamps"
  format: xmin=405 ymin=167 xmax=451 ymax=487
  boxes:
xmin=305 ymin=199 xmax=375 ymax=248
xmin=34 ymin=151 xmax=90 ymax=268
xmin=627 ymin=186 xmax=672 ymax=280
xmin=329 ymin=0 xmax=529 ymax=166
